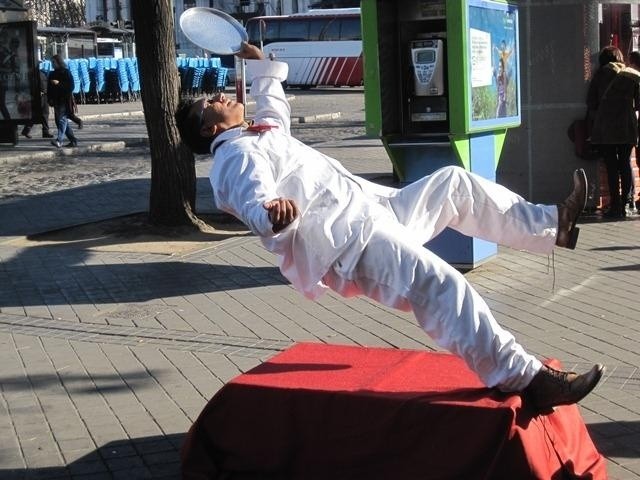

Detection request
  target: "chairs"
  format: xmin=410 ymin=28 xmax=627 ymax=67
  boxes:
xmin=38 ymin=54 xmax=229 ymax=106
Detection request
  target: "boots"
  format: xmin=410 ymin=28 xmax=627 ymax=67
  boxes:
xmin=42 ymin=133 xmax=54 ymax=137
xmin=21 ymin=130 xmax=33 ymax=138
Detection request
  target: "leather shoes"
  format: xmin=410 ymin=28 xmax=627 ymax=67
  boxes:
xmin=555 ymin=169 xmax=588 ymax=249
xmin=520 ymin=362 xmax=606 ymax=416
xmin=604 ymin=201 xmax=632 ymax=218
xmin=51 ymin=139 xmax=63 ymax=147
xmin=68 ymin=141 xmax=81 ymax=147
xmin=78 ymin=121 xmax=84 ymax=131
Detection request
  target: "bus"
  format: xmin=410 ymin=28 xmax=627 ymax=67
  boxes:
xmin=235 ymin=8 xmax=364 ymax=90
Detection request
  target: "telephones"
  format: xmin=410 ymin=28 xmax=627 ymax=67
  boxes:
xmin=403 ymin=39 xmax=444 ymax=98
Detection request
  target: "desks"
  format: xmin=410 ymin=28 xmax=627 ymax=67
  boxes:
xmin=226 ymin=341 xmax=560 ymax=480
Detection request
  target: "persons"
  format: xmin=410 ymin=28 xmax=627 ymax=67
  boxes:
xmin=491 ymin=49 xmax=515 ymax=118
xmin=174 ymin=41 xmax=606 ymax=420
xmin=585 ymin=46 xmax=640 ymax=219
xmin=496 ymin=40 xmax=514 ymax=102
xmin=67 ymin=92 xmax=83 ymax=129
xmin=21 ymin=70 xmax=54 ymax=138
xmin=46 ymin=55 xmax=77 ymax=148
xmin=3 ymin=37 xmax=24 ymax=93
xmin=0 ymin=32 xmax=13 ymax=119
xmin=627 ymin=51 xmax=640 ymax=213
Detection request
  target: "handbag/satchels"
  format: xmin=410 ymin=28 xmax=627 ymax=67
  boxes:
xmin=575 ymin=118 xmax=601 ymax=161
